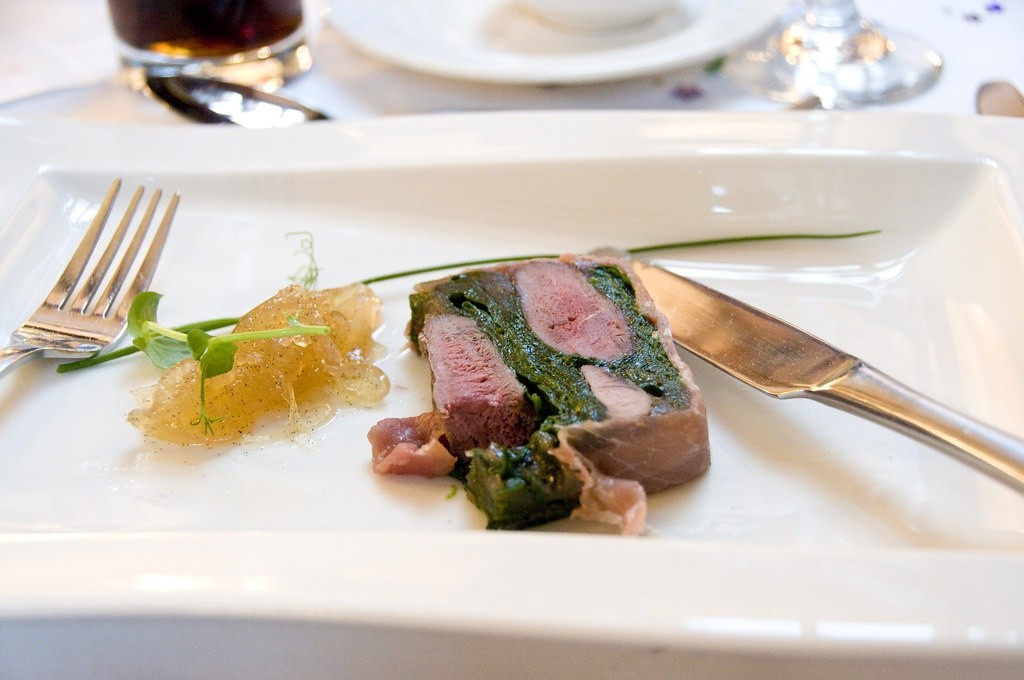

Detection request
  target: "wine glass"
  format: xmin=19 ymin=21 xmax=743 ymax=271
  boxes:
xmin=721 ymin=1 xmax=943 ymax=105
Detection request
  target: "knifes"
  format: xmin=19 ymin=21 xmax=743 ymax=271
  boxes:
xmin=630 ymin=261 xmax=1024 ymax=493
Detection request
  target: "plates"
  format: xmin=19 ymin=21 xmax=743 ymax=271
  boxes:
xmin=315 ymin=0 xmax=789 ymax=87
xmin=0 ymin=108 xmax=1024 ymax=667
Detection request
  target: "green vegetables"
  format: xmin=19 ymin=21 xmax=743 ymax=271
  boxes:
xmin=128 ymin=290 xmax=330 ymax=436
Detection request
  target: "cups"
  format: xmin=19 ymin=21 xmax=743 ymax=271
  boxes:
xmin=105 ymin=0 xmax=312 ymax=93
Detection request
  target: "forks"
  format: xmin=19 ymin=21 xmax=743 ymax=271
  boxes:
xmin=0 ymin=177 xmax=181 ymax=383
